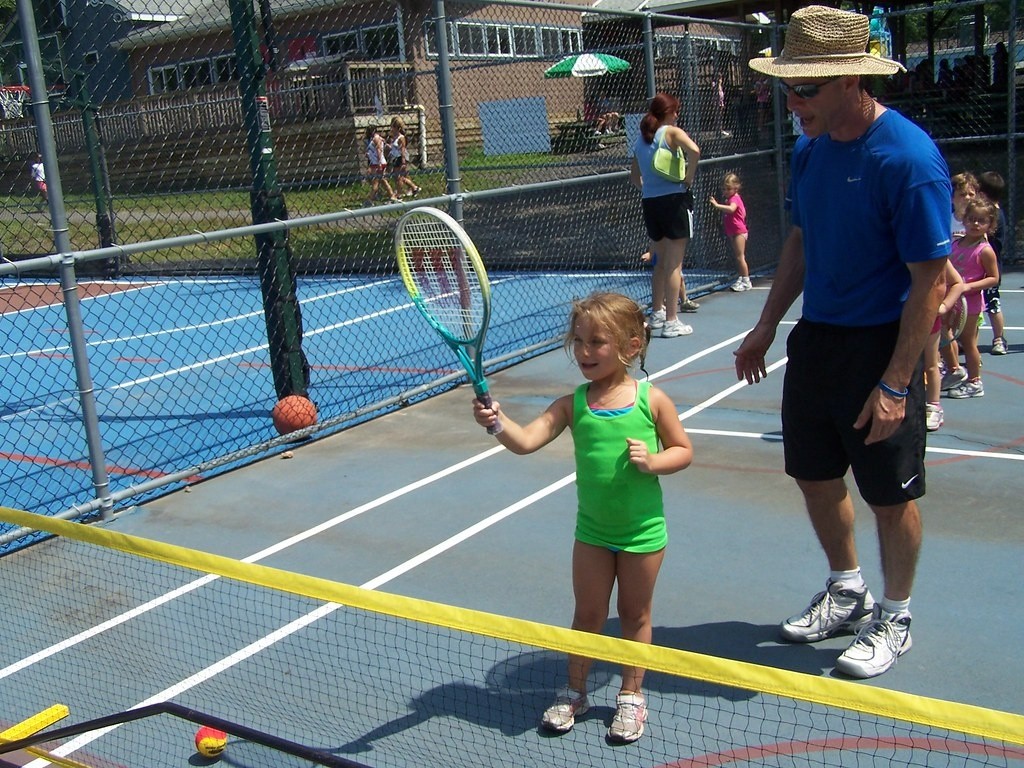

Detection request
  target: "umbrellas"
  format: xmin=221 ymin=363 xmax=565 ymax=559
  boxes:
xmin=544 ymin=54 xmax=631 ymax=79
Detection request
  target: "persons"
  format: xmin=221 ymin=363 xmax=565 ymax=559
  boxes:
xmin=359 ymin=117 xmax=422 ymax=207
xmin=926 ymin=171 xmax=1008 ymax=430
xmin=732 ymin=6 xmax=953 ymax=676
xmin=584 ymin=92 xmax=623 ymax=135
xmin=740 ymin=73 xmax=773 ymax=131
xmin=631 ymin=94 xmax=700 ymax=337
xmin=641 ymin=253 xmax=700 ymax=311
xmin=31 ymin=153 xmax=48 ymax=212
xmin=711 ymin=72 xmax=730 ymax=136
xmin=472 ymin=294 xmax=694 ymax=739
xmin=710 ymin=173 xmax=752 ymax=292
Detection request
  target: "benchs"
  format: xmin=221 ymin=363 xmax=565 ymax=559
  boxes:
xmin=551 ymin=131 xmax=627 ymax=144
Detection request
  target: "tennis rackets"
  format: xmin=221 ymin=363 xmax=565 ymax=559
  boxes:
xmin=938 ymin=294 xmax=969 ymax=349
xmin=391 ymin=206 xmax=505 ymax=437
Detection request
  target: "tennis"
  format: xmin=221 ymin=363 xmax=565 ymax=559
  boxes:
xmin=194 ymin=726 xmax=228 ymax=757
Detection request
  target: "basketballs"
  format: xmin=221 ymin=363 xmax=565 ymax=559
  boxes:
xmin=272 ymin=394 xmax=318 ymax=437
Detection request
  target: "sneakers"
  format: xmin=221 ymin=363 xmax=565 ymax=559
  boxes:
xmin=949 ymin=375 xmax=984 ymax=398
xmin=680 ymin=301 xmax=700 ymax=311
xmin=662 ymin=320 xmax=693 ymax=338
xmin=991 ymin=337 xmax=1007 ymax=355
xmin=730 ymin=276 xmax=752 ymax=291
xmin=836 ymin=602 xmax=913 ymax=678
xmin=779 ymin=577 xmax=878 ymax=642
xmin=924 ymin=402 xmax=944 ymax=431
xmin=606 ymin=692 xmax=648 ymax=740
xmin=941 ymin=362 xmax=969 ymax=390
xmin=649 ymin=308 xmax=666 ymax=329
xmin=542 ymin=685 xmax=590 ymax=732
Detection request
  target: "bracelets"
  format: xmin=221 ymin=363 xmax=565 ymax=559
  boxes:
xmin=879 ymin=382 xmax=908 ymax=397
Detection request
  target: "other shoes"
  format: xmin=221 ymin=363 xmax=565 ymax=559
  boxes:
xmin=385 ymin=197 xmax=401 ymax=205
xmin=407 ymin=187 xmax=413 ymax=196
xmin=360 ymin=200 xmax=374 ymax=207
xmin=412 ymin=185 xmax=422 ymax=198
xmin=391 ymin=192 xmax=406 ymax=199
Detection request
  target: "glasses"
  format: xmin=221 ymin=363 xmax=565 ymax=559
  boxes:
xmin=776 ymin=75 xmax=841 ymax=102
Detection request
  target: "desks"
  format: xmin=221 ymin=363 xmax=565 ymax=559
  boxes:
xmin=551 ymin=115 xmax=627 ymax=151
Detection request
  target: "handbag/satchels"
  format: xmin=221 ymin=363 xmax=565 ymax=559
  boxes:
xmin=651 ymin=127 xmax=686 ymax=184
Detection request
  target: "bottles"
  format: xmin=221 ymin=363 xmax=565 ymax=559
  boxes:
xmin=576 ymin=109 xmax=581 ymax=121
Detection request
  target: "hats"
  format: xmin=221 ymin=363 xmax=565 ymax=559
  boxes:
xmin=747 ymin=3 xmax=901 ymax=77
